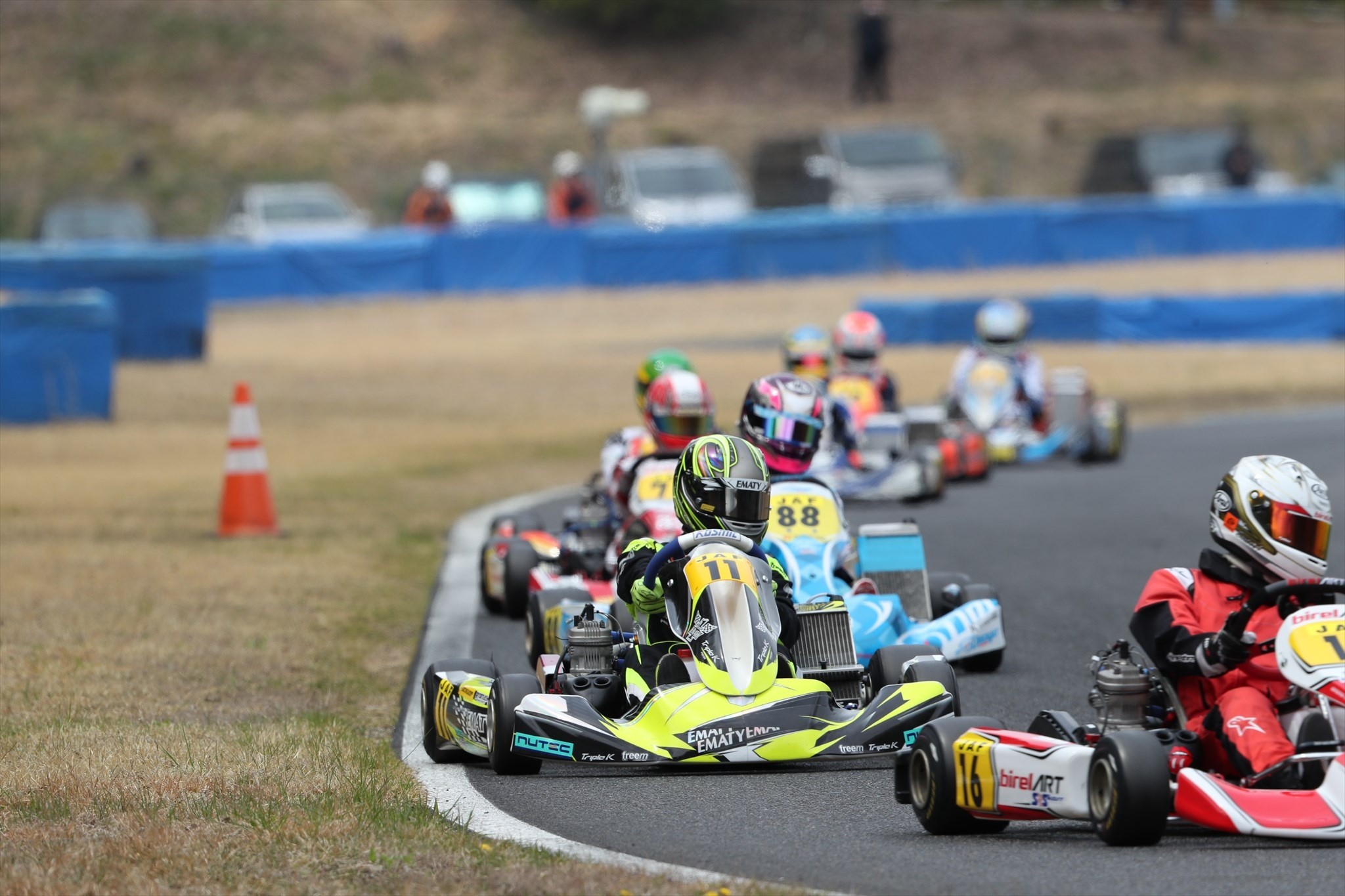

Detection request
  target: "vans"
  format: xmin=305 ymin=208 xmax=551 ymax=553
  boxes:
xmin=595 ymin=146 xmax=753 ymax=229
xmin=757 ymin=116 xmax=967 ymax=210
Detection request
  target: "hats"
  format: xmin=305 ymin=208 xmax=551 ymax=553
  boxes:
xmin=422 ymin=159 xmax=452 ymax=191
xmin=551 ymin=150 xmax=583 ymax=177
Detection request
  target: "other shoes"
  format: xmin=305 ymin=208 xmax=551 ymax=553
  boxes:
xmin=852 ymin=577 xmax=878 ymax=595
xmin=655 ymin=653 xmax=692 ymax=687
xmin=1292 ymin=713 xmax=1337 ymax=788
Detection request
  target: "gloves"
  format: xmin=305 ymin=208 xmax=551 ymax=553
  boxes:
xmin=772 ymin=579 xmax=777 ymax=598
xmin=1195 ymin=611 xmax=1256 ymax=678
xmin=630 ymin=575 xmax=666 ymax=614
xmin=630 ymin=575 xmax=667 ymax=614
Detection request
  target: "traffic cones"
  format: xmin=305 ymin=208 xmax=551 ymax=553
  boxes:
xmin=209 ymin=381 xmax=277 ymax=533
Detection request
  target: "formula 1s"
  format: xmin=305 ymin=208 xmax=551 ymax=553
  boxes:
xmin=929 ymin=350 xmax=1126 ymax=466
xmin=419 ymin=470 xmax=1012 ymax=779
xmin=809 ymin=406 xmax=990 ymax=505
xmin=888 ymin=576 xmax=1344 ymax=843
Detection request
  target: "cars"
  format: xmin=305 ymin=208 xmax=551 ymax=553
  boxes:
xmin=211 ymin=176 xmax=370 ymax=247
xmin=31 ymin=195 xmax=167 ymax=248
xmin=1076 ymin=127 xmax=1297 ymax=201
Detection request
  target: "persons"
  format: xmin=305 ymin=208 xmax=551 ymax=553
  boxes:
xmin=1226 ymin=124 xmax=1255 ymax=188
xmin=1129 ymin=458 xmax=1334 ymax=791
xmin=406 ymin=163 xmax=454 ymax=229
xmin=546 ymin=154 xmax=595 ymax=224
xmin=617 ymin=431 xmax=799 ymax=707
xmin=591 ymin=302 xmax=1054 ymax=594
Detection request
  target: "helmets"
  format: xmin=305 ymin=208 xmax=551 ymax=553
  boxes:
xmin=783 ymin=326 xmax=833 ymax=385
xmin=973 ymin=296 xmax=1031 ymax=356
xmin=742 ymin=372 xmax=824 ymax=475
xmin=645 ymin=370 xmax=708 ymax=451
xmin=832 ymin=310 xmax=884 ymax=372
xmin=1209 ymin=455 xmax=1332 ymax=580
xmin=672 ymin=434 xmax=771 ymax=546
xmin=634 ymin=350 xmax=699 ymax=414
